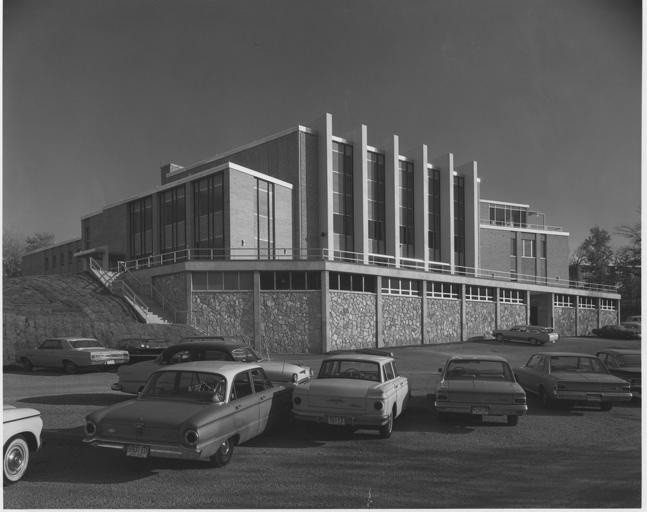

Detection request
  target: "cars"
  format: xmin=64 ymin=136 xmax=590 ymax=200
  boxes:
xmin=591 ymin=316 xmax=640 ymax=340
xmin=596 ymin=348 xmax=640 ymax=402
xmin=3 ymin=401 xmax=46 ymax=484
xmin=432 ymin=353 xmax=528 ymax=429
xmin=492 ymin=324 xmax=559 ymax=346
xmin=513 ymin=350 xmax=633 ymax=412
xmin=290 ymin=348 xmax=411 ymax=442
xmin=81 ymin=358 xmax=289 ymax=465
xmin=14 ymin=334 xmax=312 ymax=405
